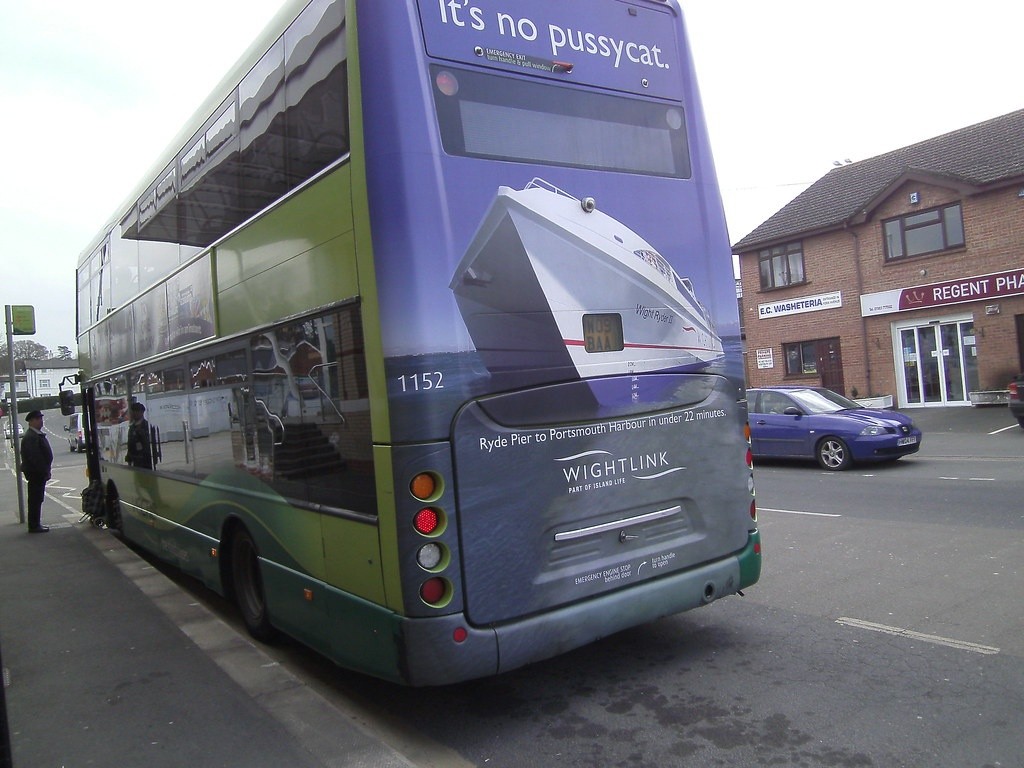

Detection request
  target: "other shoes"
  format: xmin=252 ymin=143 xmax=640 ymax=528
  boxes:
xmin=29 ymin=524 xmax=49 ymax=532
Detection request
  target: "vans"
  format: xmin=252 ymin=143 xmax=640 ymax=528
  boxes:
xmin=4 ymin=390 xmax=33 ymax=406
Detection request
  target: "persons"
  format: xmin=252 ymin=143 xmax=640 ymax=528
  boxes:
xmin=125 ymin=402 xmax=156 ymax=470
xmin=21 ymin=411 xmax=53 ymax=533
xmin=769 ymin=397 xmax=788 ymax=414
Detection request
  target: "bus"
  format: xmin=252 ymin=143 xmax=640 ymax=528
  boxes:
xmin=58 ymin=2 xmax=764 ymax=688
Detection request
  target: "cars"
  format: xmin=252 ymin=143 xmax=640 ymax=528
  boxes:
xmin=5 ymin=424 xmax=24 ymax=438
xmin=746 ymin=386 xmax=923 ymax=471
xmin=1007 ymin=374 xmax=1024 ymax=431
xmin=62 ymin=414 xmax=94 ymax=452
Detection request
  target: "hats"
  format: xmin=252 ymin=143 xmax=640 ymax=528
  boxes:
xmin=25 ymin=409 xmax=44 ymax=421
xmin=130 ymin=402 xmax=145 ymax=411
